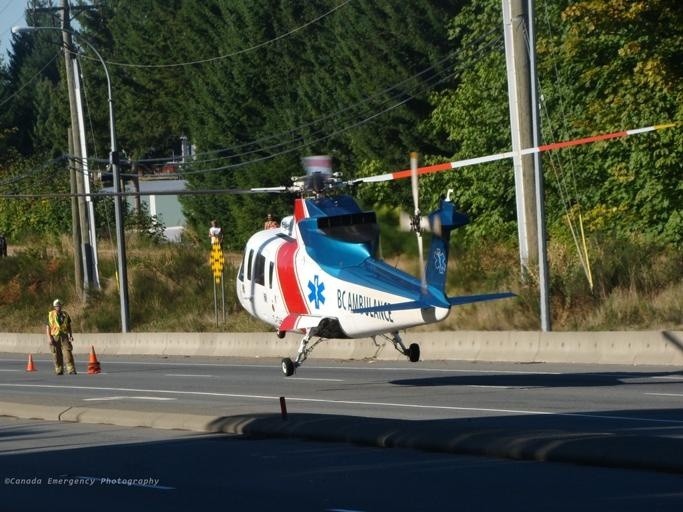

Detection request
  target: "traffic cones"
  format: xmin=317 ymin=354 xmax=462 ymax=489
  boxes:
xmin=26 ymin=354 xmax=37 ymax=371
xmin=87 ymin=344 xmax=101 ymax=374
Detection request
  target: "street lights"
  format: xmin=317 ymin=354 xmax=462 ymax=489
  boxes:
xmin=12 ymin=25 xmax=130 ymax=333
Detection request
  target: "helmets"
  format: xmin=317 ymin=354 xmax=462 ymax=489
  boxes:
xmin=53 ymin=299 xmax=61 ymax=307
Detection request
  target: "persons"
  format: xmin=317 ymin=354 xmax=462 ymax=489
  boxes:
xmin=264 ymin=214 xmax=279 ymax=230
xmin=208 ymin=220 xmax=224 ymax=250
xmin=46 ymin=299 xmax=77 ymax=374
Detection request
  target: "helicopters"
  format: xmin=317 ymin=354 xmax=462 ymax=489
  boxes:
xmin=2 ymin=124 xmax=678 ymax=375
